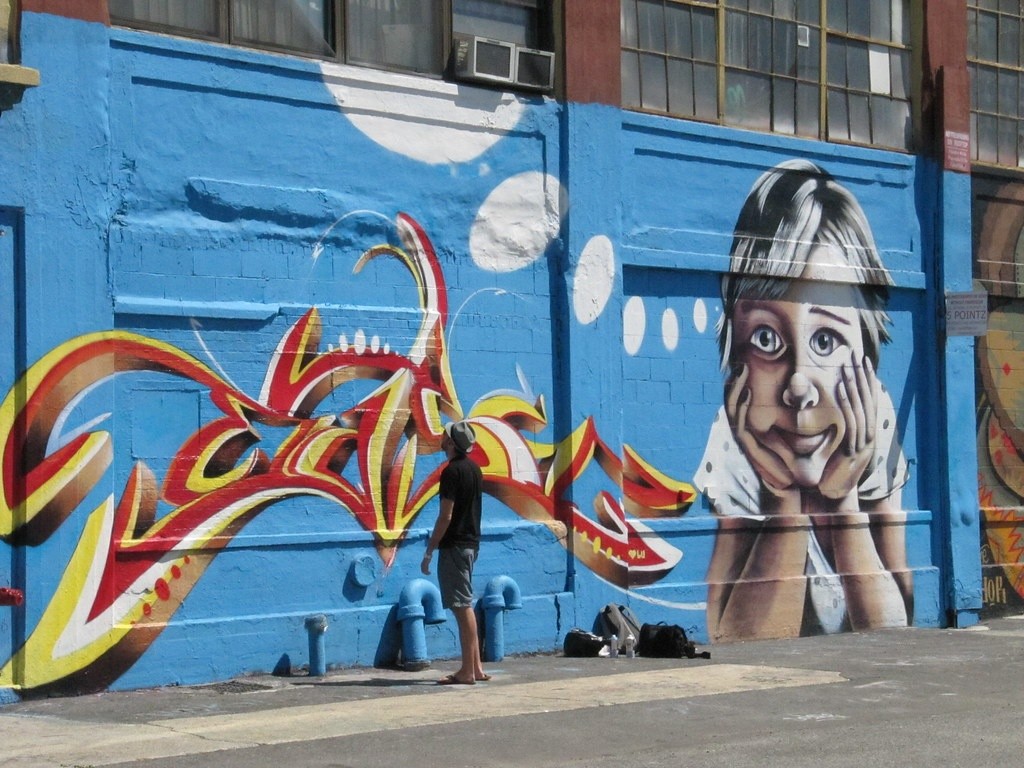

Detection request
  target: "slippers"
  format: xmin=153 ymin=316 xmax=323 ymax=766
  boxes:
xmin=438 ymin=675 xmax=477 ymax=684
xmin=475 ymin=674 xmax=491 ymax=682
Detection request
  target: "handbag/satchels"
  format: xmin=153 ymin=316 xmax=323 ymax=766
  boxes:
xmin=640 ymin=621 xmax=688 ymax=658
xmin=563 ymin=628 xmax=606 ymax=657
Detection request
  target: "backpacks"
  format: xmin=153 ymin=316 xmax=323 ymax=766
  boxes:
xmin=600 ymin=603 xmax=643 ymax=650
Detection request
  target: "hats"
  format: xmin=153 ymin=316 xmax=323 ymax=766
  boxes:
xmin=444 ymin=419 xmax=478 ymax=455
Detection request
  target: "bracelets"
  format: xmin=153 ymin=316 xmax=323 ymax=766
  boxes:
xmin=423 ymin=553 xmax=432 ymax=560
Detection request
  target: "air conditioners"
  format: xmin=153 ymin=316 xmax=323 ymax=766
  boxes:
xmin=513 ymin=47 xmax=555 ymax=93
xmin=456 ymin=35 xmax=515 ymax=84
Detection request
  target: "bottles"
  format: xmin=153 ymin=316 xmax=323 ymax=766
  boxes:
xmin=611 ymin=635 xmax=618 ymax=658
xmin=625 ymin=634 xmax=635 ymax=658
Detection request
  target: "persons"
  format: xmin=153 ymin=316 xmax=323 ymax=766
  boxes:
xmin=421 ymin=421 xmax=492 ymax=684
